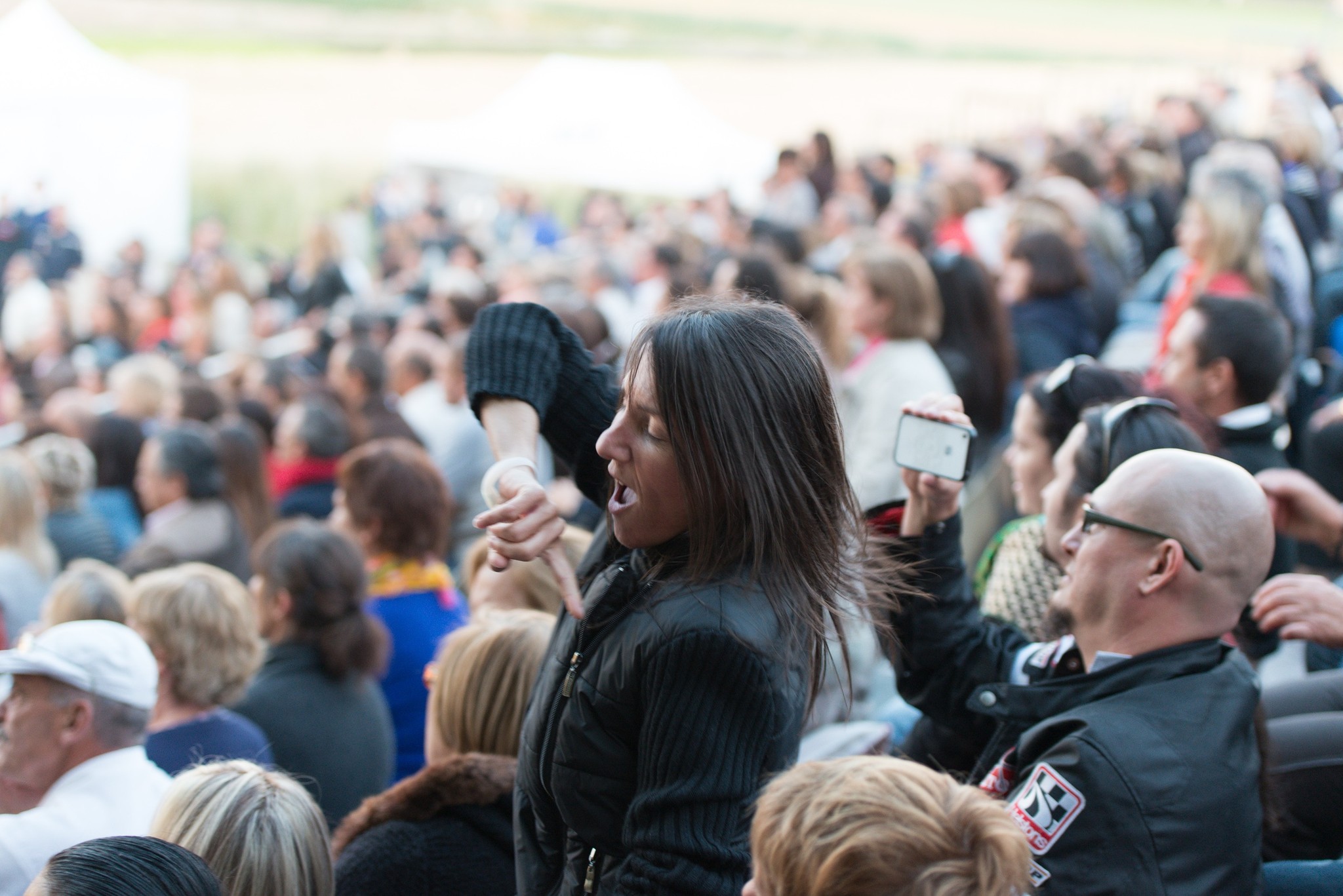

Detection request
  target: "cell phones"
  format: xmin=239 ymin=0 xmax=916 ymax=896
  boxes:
xmin=895 ymin=415 xmax=978 ymax=482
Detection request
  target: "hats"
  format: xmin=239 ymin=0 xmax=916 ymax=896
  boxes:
xmin=29 ymin=432 xmax=94 ymax=487
xmin=0 ymin=621 xmax=160 ymax=709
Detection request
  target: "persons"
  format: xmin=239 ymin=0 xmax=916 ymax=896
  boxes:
xmin=0 ymin=56 xmax=1343 ymax=896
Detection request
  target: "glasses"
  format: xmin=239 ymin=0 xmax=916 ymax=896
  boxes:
xmin=1099 ymin=395 xmax=1179 ymax=472
xmin=1079 ymin=504 xmax=1207 ymax=573
xmin=424 ymin=664 xmax=437 ymax=690
xmin=1044 ymin=356 xmax=1103 ymax=419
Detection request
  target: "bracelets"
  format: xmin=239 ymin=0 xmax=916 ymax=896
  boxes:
xmin=480 ymin=456 xmax=536 ymax=511
